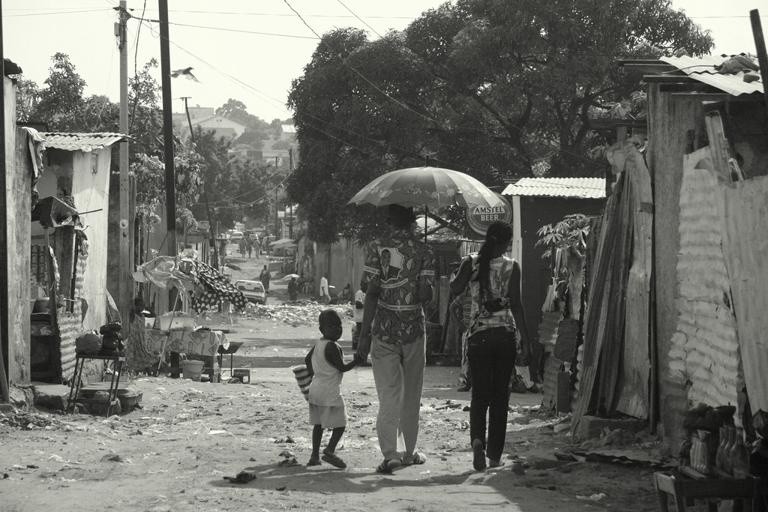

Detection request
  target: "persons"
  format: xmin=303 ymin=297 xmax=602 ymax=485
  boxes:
xmin=287 ymin=278 xmax=297 ymax=300
xmin=261 ymin=264 xmax=271 ymax=293
xmin=305 ymin=308 xmax=362 ymax=470
xmin=320 ymin=273 xmax=331 ymax=303
xmin=361 ymin=202 xmax=437 ymax=474
xmin=449 ymin=221 xmax=534 ymax=469
xmin=238 ymin=235 xmax=261 ymax=260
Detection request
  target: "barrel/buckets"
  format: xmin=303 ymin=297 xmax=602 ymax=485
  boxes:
xmin=183 ymin=359 xmax=205 ymax=382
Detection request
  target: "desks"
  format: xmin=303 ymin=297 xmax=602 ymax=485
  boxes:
xmin=64 ymin=346 xmax=128 ymax=421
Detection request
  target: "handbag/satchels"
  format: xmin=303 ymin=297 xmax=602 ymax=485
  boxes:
xmin=293 ymin=367 xmax=314 ymax=400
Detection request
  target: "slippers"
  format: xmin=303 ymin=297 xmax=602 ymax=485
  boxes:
xmin=306 ymin=454 xmax=347 ymax=470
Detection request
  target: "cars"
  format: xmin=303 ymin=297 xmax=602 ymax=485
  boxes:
xmin=231 ymin=232 xmax=244 ymax=243
xmin=235 ymin=280 xmax=267 ymax=304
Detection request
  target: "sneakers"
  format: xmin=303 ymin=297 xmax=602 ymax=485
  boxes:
xmin=457 ymin=375 xmax=472 ymax=391
xmin=378 ymin=458 xmax=401 ymax=473
xmin=488 ymin=458 xmax=505 ymax=468
xmin=514 ymin=377 xmax=527 ymax=393
xmin=403 ymin=452 xmax=426 ymax=464
xmin=473 ymin=439 xmax=486 ymax=470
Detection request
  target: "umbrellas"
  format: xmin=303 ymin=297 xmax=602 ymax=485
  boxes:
xmin=281 ymin=273 xmax=300 ymax=281
xmin=345 ymin=157 xmax=503 ymax=236
